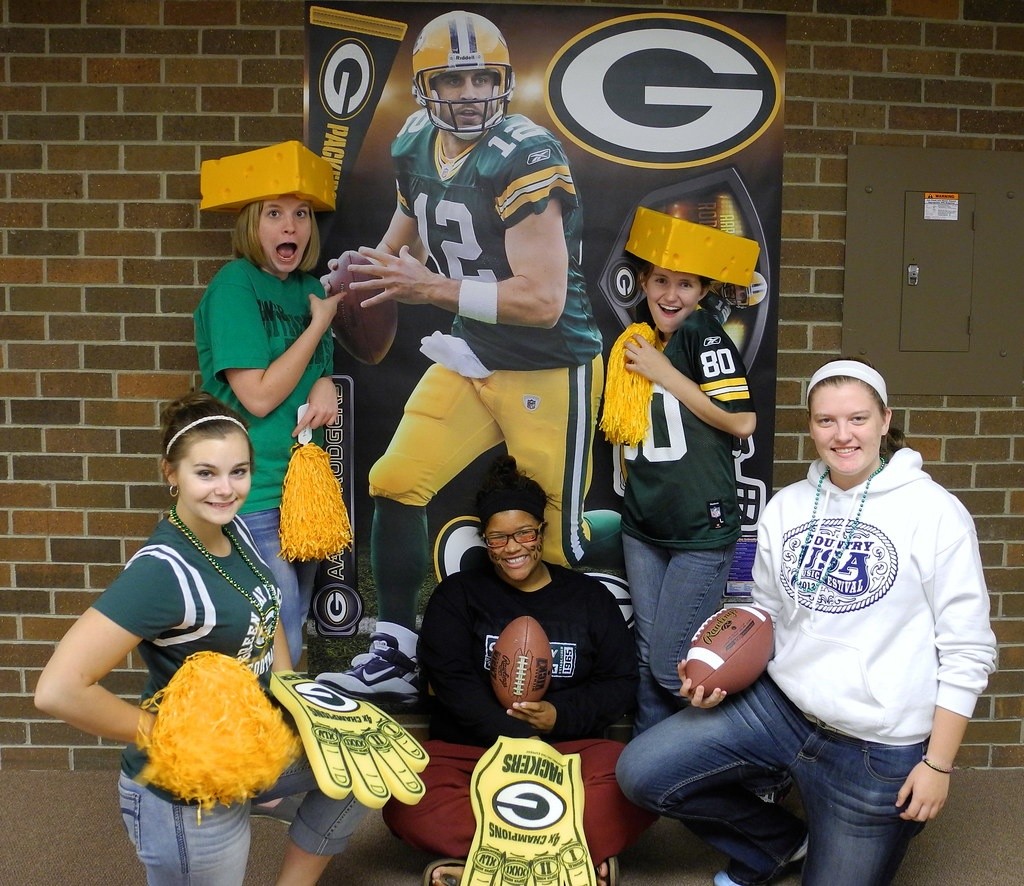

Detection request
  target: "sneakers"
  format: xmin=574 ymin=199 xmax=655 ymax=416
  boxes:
xmin=314 ymin=621 xmax=419 ymax=706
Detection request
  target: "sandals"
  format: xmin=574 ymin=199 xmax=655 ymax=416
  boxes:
xmin=421 ymin=858 xmax=465 ymax=886
xmin=595 ymin=856 xmax=621 ymax=886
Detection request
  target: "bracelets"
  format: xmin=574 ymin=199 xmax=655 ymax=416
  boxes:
xmin=457 ymin=279 xmax=497 ymax=326
xmin=923 ymin=756 xmax=953 ymax=774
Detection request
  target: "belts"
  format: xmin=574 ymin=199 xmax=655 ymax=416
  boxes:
xmin=803 ymin=714 xmax=859 ymax=738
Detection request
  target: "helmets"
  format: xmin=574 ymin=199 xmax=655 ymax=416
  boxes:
xmin=412 ymin=11 xmax=514 ymax=140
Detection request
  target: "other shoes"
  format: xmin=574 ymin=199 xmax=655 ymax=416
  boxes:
xmin=250 ymin=798 xmax=304 ymax=824
xmin=713 ymin=834 xmax=808 ymax=886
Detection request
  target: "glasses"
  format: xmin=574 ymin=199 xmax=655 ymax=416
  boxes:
xmin=482 ymin=522 xmax=543 ymax=548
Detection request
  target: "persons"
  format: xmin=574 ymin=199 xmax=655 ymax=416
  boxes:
xmin=316 ymin=11 xmax=622 ymax=708
xmin=195 ymin=193 xmax=347 ymax=826
xmin=382 ymin=456 xmax=658 ymax=886
xmin=620 ymin=263 xmax=757 ymax=739
xmin=615 ymin=355 xmax=996 ymax=886
xmin=35 ymin=391 xmax=373 ymax=886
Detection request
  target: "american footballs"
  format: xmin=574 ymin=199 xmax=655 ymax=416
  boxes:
xmin=328 ymin=247 xmax=401 ymax=367
xmin=488 ymin=614 xmax=554 ymax=712
xmin=684 ymin=605 xmax=777 ymax=698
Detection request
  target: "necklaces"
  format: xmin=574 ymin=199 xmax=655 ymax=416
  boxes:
xmin=169 ymin=504 xmax=280 ymax=644
xmin=794 ymin=456 xmax=885 ymax=591
